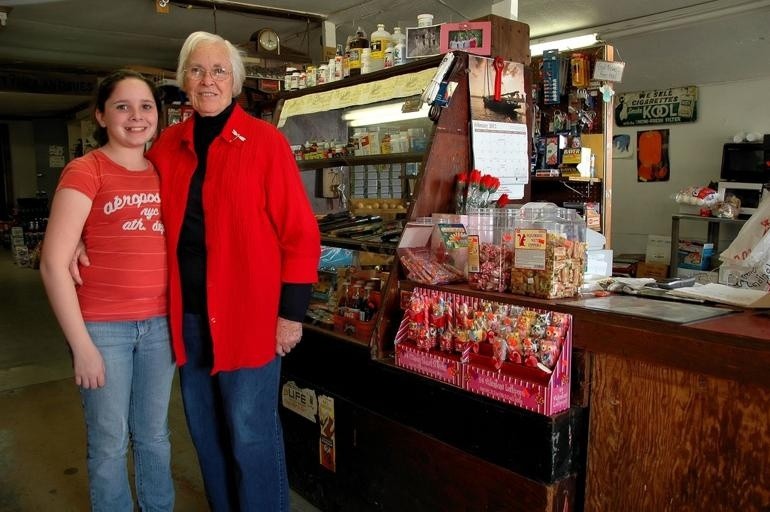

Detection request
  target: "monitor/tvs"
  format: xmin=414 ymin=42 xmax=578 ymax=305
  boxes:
xmin=720 ymin=142 xmax=770 ymax=184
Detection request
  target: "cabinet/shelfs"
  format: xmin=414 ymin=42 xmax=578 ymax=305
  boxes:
xmin=263 ymin=45 xmax=615 ymax=511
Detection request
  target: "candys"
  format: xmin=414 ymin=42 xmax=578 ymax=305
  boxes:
xmin=399 ymin=232 xmax=586 ymax=373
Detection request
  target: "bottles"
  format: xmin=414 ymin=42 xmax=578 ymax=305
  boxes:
xmin=351 ymin=283 xmax=361 ymax=318
xmin=282 ymin=136 xmax=348 ymax=165
xmin=349 ymin=23 xmax=405 ymax=76
xmin=348 ymin=127 xmax=428 ymax=157
xmin=338 ymin=281 xmax=350 ymax=316
xmin=284 ymin=44 xmax=344 ymax=92
xmin=362 ymin=286 xmax=376 ymax=322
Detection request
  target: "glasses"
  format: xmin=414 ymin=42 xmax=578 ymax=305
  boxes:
xmin=181 ymin=65 xmax=233 ymax=82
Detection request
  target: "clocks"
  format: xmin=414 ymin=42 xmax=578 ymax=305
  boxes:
xmin=257 ymin=28 xmax=280 ymax=57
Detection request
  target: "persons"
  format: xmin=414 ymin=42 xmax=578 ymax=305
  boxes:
xmin=40 ymin=70 xmax=174 ymax=511
xmin=450 ymin=33 xmax=477 ymax=50
xmin=70 ymin=31 xmax=321 ymax=511
xmin=414 ymin=29 xmax=435 ymax=50
